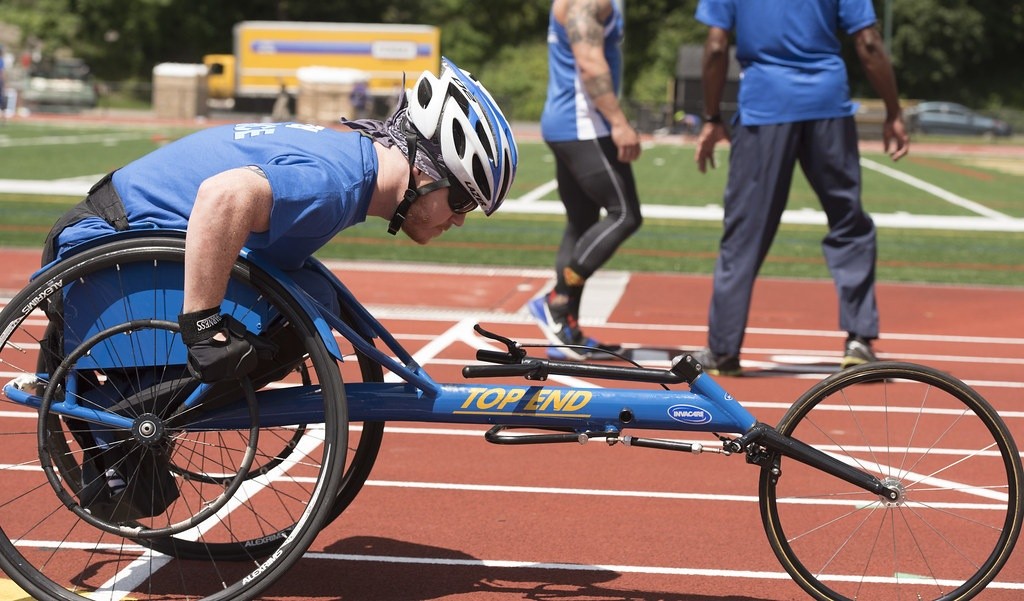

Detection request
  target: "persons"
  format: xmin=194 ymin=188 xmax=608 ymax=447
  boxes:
xmin=527 ymin=0 xmax=644 ymax=361
xmin=692 ymin=0 xmax=910 ymax=381
xmin=38 ymin=54 xmax=522 ymax=386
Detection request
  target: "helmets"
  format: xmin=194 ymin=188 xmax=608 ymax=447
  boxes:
xmin=404 ymin=55 xmax=519 ymax=216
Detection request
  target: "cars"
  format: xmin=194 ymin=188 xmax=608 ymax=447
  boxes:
xmin=912 ymin=102 xmax=1012 ymax=143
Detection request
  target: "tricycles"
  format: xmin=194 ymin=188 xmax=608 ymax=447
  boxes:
xmin=0 ymin=226 xmax=1024 ymax=600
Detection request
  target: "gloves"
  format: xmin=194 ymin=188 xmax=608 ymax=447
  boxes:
xmin=177 ymin=305 xmax=282 ymax=385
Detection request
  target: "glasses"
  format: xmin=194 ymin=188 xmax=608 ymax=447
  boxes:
xmin=447 ymin=172 xmax=479 ymax=214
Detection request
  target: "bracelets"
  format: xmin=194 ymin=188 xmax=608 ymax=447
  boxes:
xmin=703 ymin=112 xmax=725 ymax=123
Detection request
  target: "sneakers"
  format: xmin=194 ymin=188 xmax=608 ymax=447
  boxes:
xmin=670 ymin=345 xmax=743 ymax=376
xmin=841 ymin=336 xmax=893 ymax=383
xmin=528 ymin=293 xmax=623 ymax=361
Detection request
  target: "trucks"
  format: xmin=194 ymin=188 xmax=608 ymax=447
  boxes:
xmin=203 ymin=19 xmax=441 ymax=120
